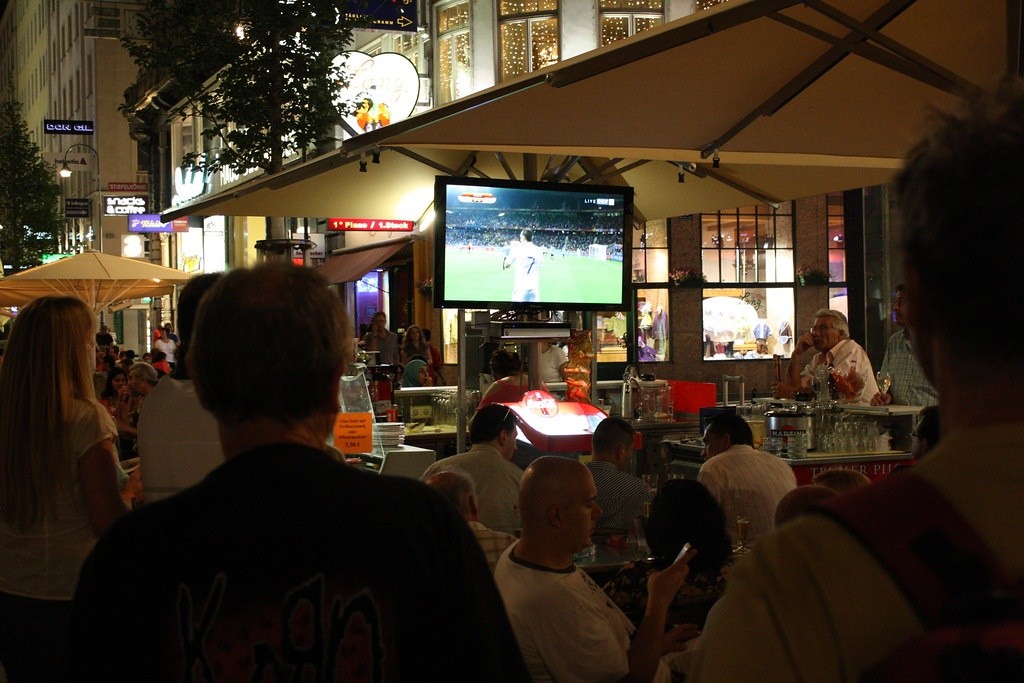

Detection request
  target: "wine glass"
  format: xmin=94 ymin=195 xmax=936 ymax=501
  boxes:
xmin=876 ymin=370 xmax=891 ymax=408
xmin=815 ymin=409 xmax=891 ymax=454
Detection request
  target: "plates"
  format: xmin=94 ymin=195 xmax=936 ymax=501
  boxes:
xmin=119 ymin=455 xmax=142 ymax=474
xmin=370 ymin=422 xmax=406 ymax=446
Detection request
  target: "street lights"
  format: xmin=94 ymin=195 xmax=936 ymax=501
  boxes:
xmin=60 ymin=145 xmax=104 ymax=327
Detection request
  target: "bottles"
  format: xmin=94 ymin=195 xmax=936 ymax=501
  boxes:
xmin=811 ymin=360 xmax=865 ymax=407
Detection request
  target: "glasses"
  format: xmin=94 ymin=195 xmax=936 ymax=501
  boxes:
xmin=502 ymin=406 xmax=512 ymax=423
xmin=809 ymin=326 xmax=832 ymax=335
xmin=908 ymin=431 xmax=922 ymax=439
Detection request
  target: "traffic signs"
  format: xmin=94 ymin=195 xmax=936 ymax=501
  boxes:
xmin=65 ymin=197 xmax=90 ymax=218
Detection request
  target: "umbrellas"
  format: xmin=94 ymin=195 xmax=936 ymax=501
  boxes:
xmin=0 ymin=253 xmax=194 ymax=370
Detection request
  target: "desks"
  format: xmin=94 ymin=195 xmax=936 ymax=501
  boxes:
xmin=568 ymin=531 xmax=644 ymax=573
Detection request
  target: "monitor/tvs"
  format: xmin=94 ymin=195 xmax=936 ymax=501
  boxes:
xmin=432 ymin=175 xmax=635 ymax=312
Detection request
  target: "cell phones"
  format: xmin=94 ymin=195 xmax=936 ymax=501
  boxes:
xmin=672 ymin=543 xmax=692 ymax=566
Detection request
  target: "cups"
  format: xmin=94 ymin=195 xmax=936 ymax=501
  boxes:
xmin=432 ymin=389 xmax=481 ymax=427
xmin=786 ymin=433 xmax=809 ymax=459
xmin=763 ymin=436 xmax=783 ymax=460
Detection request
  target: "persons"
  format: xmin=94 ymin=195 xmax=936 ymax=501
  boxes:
xmin=0 ymin=297 xmax=141 ymax=683
xmin=93 ymin=271 xmax=938 ymax=683
xmin=688 ymin=77 xmax=1024 ymax=683
xmin=448 ymin=213 xmax=623 ymax=303
xmin=72 ymin=262 xmax=533 ymax=683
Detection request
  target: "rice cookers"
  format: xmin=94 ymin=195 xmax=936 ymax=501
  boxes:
xmin=764 ymin=411 xmax=820 ymax=451
